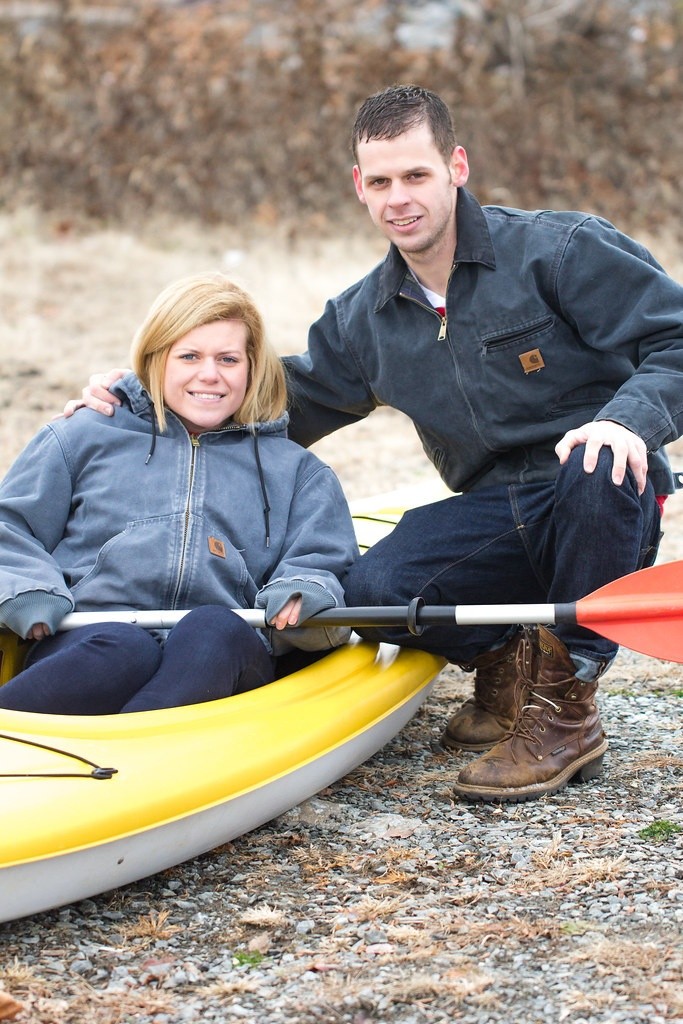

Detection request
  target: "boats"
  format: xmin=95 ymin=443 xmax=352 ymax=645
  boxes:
xmin=0 ymin=505 xmax=450 ymax=926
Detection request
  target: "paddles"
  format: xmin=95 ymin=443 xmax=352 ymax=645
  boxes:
xmin=60 ymin=561 xmax=681 ymax=669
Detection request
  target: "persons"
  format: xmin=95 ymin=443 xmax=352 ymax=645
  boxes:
xmin=53 ymin=86 xmax=683 ymax=797
xmin=1 ymin=275 xmax=361 ymax=715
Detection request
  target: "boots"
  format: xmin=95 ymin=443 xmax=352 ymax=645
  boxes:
xmin=441 ymin=623 xmax=534 ymax=753
xmin=453 ymin=624 xmax=609 ymax=803
